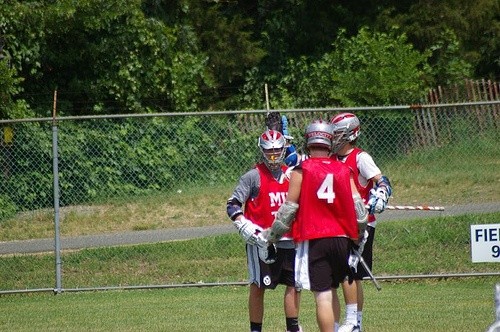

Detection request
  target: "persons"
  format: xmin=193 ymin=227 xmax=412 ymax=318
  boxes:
xmin=257 ymin=117 xmax=369 ymax=332
xmin=226 ymin=130 xmax=303 ymax=332
xmin=226 ymin=110 xmax=392 ymax=332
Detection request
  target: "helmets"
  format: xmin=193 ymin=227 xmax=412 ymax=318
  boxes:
xmin=306 ymin=117 xmax=333 ymax=150
xmin=257 ymin=130 xmax=285 ymax=171
xmin=330 ymin=113 xmax=360 ymax=152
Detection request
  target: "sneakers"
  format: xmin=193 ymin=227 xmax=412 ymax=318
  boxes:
xmin=337 ymin=322 xmax=360 ymax=332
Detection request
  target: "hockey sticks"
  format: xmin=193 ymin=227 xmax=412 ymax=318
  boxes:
xmin=364 ymin=204 xmax=447 ymax=212
xmin=348 ymin=244 xmax=383 ymax=293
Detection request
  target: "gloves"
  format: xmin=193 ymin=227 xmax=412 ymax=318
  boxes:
xmin=366 ymin=188 xmax=389 ymax=214
xmin=257 ymin=233 xmax=277 ymax=264
xmin=265 ymin=111 xmax=293 ymax=142
xmin=233 ymin=216 xmax=263 ymax=246
